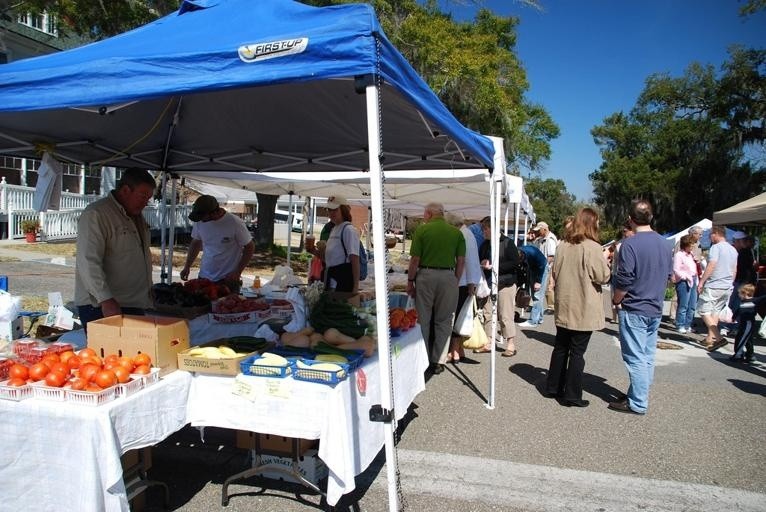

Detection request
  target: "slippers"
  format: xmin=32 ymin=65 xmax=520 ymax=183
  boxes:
xmin=473 ymin=346 xmax=491 ymax=353
xmin=501 ymin=349 xmax=517 ymax=357
xmin=446 ymin=353 xmax=460 ymax=364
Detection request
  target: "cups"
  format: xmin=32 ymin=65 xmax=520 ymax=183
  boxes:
xmin=391 ymin=327 xmax=401 ymax=338
xmin=305 ymin=236 xmax=315 ymax=250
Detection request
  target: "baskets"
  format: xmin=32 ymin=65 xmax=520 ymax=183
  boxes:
xmin=241 ymin=346 xmax=366 ymax=384
xmin=0 ymin=367 xmax=162 ymax=407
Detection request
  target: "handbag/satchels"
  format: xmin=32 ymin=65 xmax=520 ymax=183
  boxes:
xmin=514 ymin=287 xmax=531 ymax=308
xmin=461 ymin=317 xmax=489 ymax=348
xmin=342 ymin=223 xmax=368 ymax=280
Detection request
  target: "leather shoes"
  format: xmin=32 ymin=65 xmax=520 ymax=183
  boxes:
xmin=609 ymin=394 xmax=644 ymax=414
xmin=566 ymin=399 xmax=589 ymax=407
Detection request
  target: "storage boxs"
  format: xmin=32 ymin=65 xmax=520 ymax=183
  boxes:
xmin=0 ymin=316 xmax=24 ymax=342
xmin=86 ymin=314 xmax=189 ymax=378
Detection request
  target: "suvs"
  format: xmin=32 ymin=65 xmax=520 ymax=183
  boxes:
xmin=383 ymin=228 xmax=403 ymax=243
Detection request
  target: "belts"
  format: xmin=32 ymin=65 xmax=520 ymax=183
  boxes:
xmin=418 ymin=265 xmax=456 ymax=271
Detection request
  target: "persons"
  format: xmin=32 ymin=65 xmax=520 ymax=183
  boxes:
xmin=306 ymin=197 xmax=360 ymax=294
xmin=179 ymin=195 xmax=254 ymax=284
xmin=320 ymin=205 xmax=351 ymax=282
xmin=407 ymin=200 xmax=558 ymax=375
xmin=543 ymin=200 xmax=674 ymax=415
xmin=75 ymin=167 xmax=156 ymax=339
xmin=671 ymin=224 xmax=756 ymax=363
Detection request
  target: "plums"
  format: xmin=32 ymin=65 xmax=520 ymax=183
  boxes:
xmin=213 ymin=293 xmax=270 ymax=321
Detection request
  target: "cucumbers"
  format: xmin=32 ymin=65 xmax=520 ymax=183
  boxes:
xmin=309 ymin=300 xmax=367 ymax=338
xmin=228 ymin=336 xmax=359 ymax=358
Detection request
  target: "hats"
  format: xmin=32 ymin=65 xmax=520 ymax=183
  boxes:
xmin=324 ymin=194 xmax=350 ymax=210
xmin=188 ymin=195 xmax=217 ymax=222
xmin=532 ymin=222 xmax=548 ymax=231
xmin=733 ymin=231 xmax=748 ymax=239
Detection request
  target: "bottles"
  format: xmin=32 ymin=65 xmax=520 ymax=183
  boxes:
xmin=252 ymin=275 xmax=261 ymax=291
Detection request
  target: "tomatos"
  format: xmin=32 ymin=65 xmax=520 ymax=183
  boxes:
xmin=8 ymin=347 xmax=151 ymax=392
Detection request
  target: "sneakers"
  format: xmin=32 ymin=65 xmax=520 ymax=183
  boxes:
xmin=544 ymin=309 xmax=554 ymax=315
xmin=700 ymin=338 xmax=711 ymax=345
xmin=731 ymin=351 xmax=756 ymax=362
xmin=518 ymin=320 xmax=544 ymax=327
xmin=708 ymin=338 xmax=728 ymax=352
xmin=676 ymin=327 xmax=694 ymax=334
xmin=432 ymin=362 xmax=444 ymax=374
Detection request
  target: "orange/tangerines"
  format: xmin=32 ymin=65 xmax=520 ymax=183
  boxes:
xmin=390 ymin=308 xmax=419 ymax=330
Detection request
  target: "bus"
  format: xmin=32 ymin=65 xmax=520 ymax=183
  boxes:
xmin=273 ymin=210 xmax=309 ymax=233
